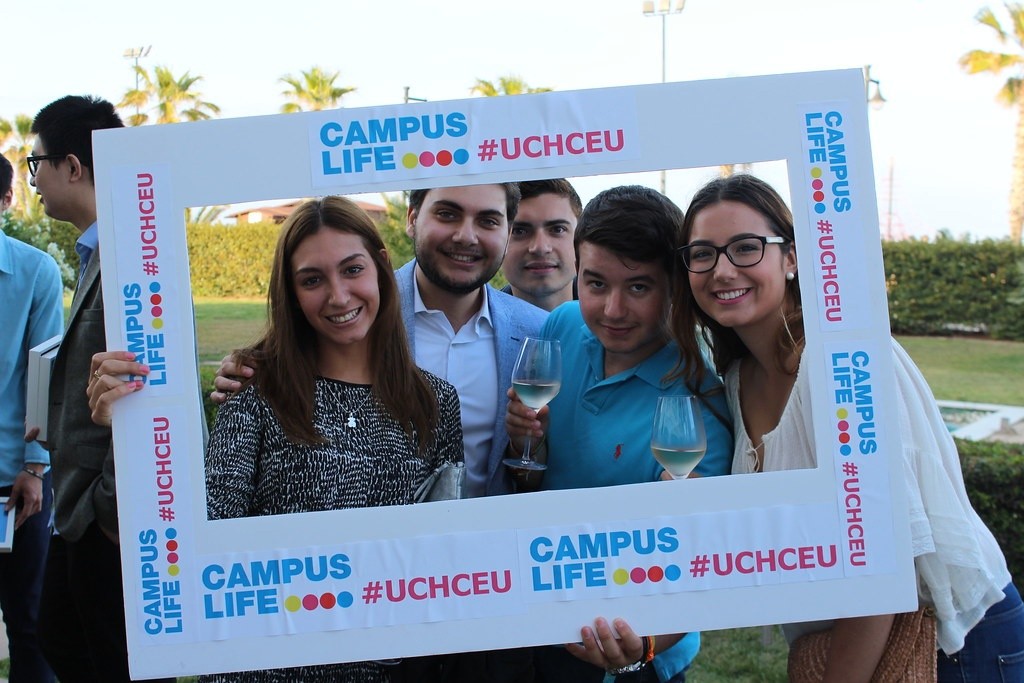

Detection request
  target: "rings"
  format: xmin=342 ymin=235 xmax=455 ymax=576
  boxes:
xmin=92 ymin=369 xmax=102 ymax=379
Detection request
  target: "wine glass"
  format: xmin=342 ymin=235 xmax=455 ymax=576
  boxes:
xmin=502 ymin=336 xmax=562 ymax=471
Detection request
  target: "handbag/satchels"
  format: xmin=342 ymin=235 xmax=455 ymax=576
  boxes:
xmin=788 ymin=605 xmax=942 ymax=683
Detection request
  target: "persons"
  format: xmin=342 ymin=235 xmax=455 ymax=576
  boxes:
xmin=86 ymin=191 xmax=469 ymax=522
xmin=678 ymin=174 xmax=1024 ymax=681
xmin=504 ymin=178 xmax=593 ymax=311
xmin=205 ymin=179 xmax=554 ymax=504
xmin=497 ymin=185 xmax=734 ymax=683
xmin=0 ymin=153 xmax=71 ymax=683
xmin=20 ymin=97 xmax=138 ymax=681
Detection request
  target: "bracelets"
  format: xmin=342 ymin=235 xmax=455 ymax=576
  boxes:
xmin=605 ymin=638 xmax=654 ymax=675
xmin=24 ymin=467 xmax=46 ymax=481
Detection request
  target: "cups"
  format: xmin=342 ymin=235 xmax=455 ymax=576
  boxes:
xmin=653 ymin=395 xmax=706 ymax=480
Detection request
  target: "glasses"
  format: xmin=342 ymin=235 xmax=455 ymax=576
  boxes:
xmin=26 ymin=153 xmax=67 ymax=177
xmin=679 ymin=236 xmax=788 ymax=274
